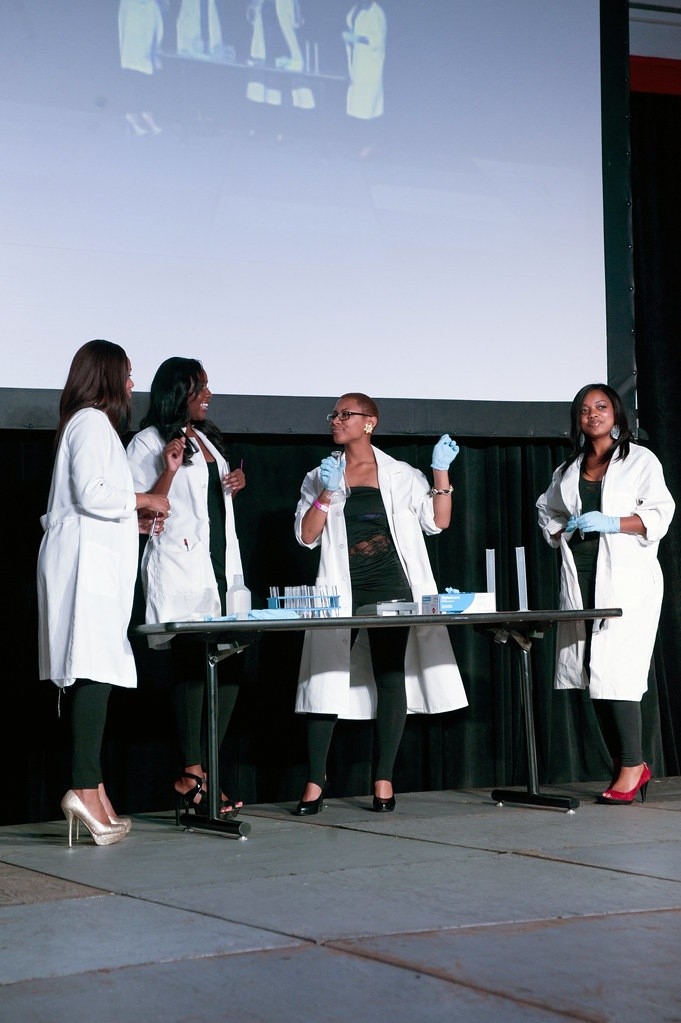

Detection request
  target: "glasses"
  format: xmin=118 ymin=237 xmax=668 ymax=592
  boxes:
xmin=326 ymin=409 xmax=371 ymax=423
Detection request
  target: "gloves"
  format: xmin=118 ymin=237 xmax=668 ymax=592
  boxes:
xmin=320 ymin=456 xmax=346 ymax=492
xmin=430 ymin=434 xmax=460 ymax=471
xmin=577 ymin=511 xmax=621 ymax=534
xmin=564 ymin=516 xmax=580 ymax=532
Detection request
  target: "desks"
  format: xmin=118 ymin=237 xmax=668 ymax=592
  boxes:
xmin=130 ymin=608 xmax=624 ymax=841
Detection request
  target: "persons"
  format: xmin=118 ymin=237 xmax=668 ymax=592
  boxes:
xmin=535 ymin=383 xmax=675 ymax=804
xmin=293 ymin=393 xmax=470 ymax=815
xmin=118 ymin=0 xmax=387 ymax=155
xmin=125 ymin=357 xmax=250 ymax=825
xmin=37 ymin=338 xmax=138 ymax=850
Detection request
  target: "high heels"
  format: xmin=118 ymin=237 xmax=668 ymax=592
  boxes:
xmin=175 ymin=772 xmax=242 ymax=826
xmin=597 ymin=761 xmax=652 ymax=805
xmin=296 ymin=780 xmax=330 ymax=815
xmin=61 ymin=789 xmax=132 ymax=848
xmin=373 ymin=784 xmax=396 ymax=811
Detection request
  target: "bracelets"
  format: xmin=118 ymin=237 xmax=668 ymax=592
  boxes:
xmin=314 ymin=500 xmax=328 ymax=512
xmin=430 ymin=486 xmax=453 ymax=496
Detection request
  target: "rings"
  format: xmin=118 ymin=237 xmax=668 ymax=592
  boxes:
xmin=167 ymin=509 xmax=172 ymax=516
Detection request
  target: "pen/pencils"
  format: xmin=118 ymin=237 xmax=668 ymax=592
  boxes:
xmin=184 ymin=538 xmax=189 ymax=551
xmin=149 ymin=512 xmax=158 ymax=539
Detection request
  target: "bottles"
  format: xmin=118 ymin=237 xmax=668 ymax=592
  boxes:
xmin=226 ymin=574 xmax=252 ymax=621
xmin=327 ymin=450 xmax=352 ymax=503
